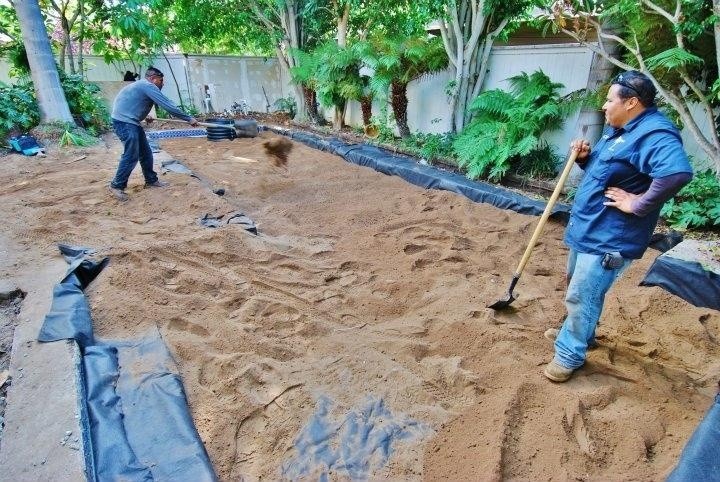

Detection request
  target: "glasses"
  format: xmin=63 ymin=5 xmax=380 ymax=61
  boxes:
xmin=611 ymin=74 xmax=642 ymax=98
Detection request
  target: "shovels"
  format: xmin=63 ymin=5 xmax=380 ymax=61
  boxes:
xmin=145 ymin=117 xmax=259 ymax=138
xmin=488 ymin=147 xmax=580 ymax=311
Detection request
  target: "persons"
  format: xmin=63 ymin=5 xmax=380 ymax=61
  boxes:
xmin=541 ymin=69 xmax=695 ymax=384
xmin=108 ymin=67 xmax=199 ymax=204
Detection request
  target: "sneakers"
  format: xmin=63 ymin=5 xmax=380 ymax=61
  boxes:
xmin=145 ymin=178 xmax=170 ymax=189
xmin=544 ymin=356 xmax=577 ymax=382
xmin=106 ymin=184 xmax=131 ymax=203
xmin=544 ymin=328 xmax=595 ymax=350
